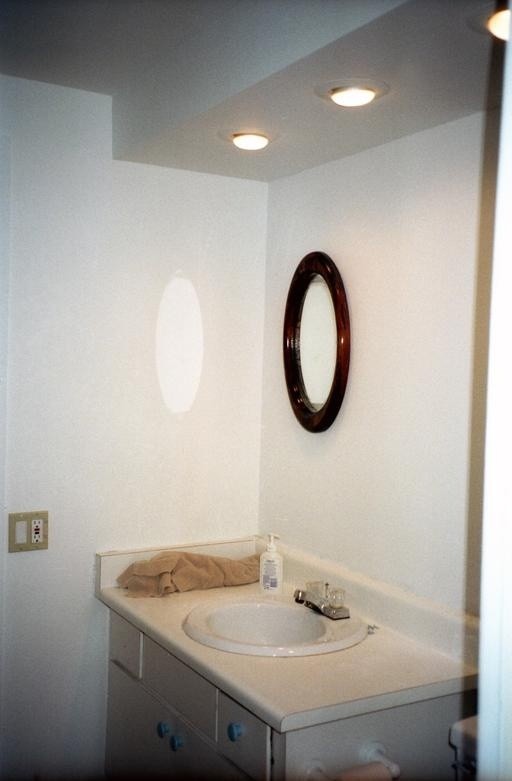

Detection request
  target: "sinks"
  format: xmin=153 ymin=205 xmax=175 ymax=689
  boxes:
xmin=183 ymin=598 xmax=369 ymax=656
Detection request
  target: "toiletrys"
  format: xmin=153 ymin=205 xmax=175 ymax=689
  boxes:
xmin=258 ymin=530 xmax=286 ymax=595
xmin=259 ymin=533 xmax=283 ymax=593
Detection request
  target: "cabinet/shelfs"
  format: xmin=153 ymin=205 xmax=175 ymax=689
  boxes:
xmin=107 ymin=610 xmax=478 ymax=781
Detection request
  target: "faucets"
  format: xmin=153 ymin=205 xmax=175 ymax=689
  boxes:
xmin=293 ymin=581 xmax=350 ymax=619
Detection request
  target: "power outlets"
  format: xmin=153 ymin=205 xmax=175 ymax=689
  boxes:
xmin=30 ymin=511 xmax=48 ymax=552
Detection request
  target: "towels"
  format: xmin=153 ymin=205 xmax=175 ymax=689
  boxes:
xmin=118 ymin=552 xmax=263 ymax=599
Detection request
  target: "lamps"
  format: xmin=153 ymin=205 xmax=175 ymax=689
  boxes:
xmin=219 ymin=127 xmax=279 ymax=152
xmin=314 ymin=78 xmax=389 ymax=107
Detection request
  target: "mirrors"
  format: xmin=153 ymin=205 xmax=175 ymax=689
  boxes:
xmin=281 ymin=250 xmax=351 ymax=431
